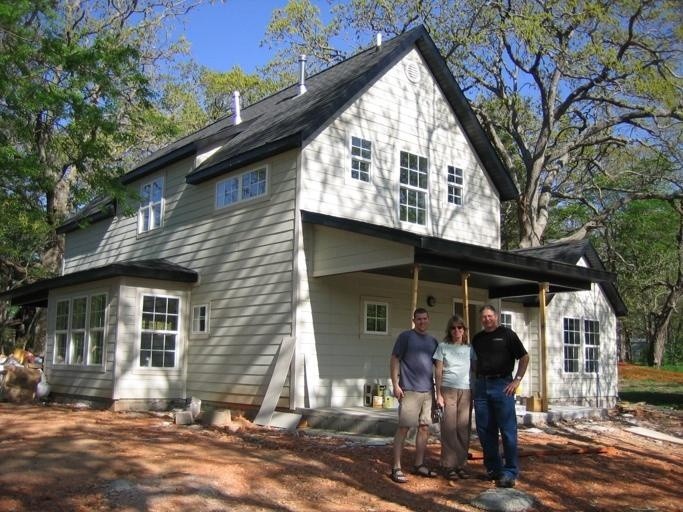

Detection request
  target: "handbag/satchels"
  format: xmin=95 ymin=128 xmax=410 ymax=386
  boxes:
xmin=432 ymin=404 xmax=444 ymax=424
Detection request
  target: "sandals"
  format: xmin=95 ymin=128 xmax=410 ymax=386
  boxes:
xmin=390 ymin=468 xmax=408 ymax=483
xmin=412 ymin=464 xmax=437 ymax=477
xmin=442 ymin=466 xmax=471 ymax=480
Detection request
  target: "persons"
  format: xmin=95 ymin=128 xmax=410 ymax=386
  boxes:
xmin=389 ymin=307 xmax=439 ymax=482
xmin=470 ymin=304 xmax=531 ymax=488
xmin=432 ymin=314 xmax=477 ymax=481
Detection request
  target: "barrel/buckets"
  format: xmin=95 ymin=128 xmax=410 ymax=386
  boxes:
xmin=373 ymin=395 xmax=383 ymax=409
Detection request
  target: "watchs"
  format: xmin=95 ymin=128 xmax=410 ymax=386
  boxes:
xmin=515 ymin=375 xmax=522 ymax=380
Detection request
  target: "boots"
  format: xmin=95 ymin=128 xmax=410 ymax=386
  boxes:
xmin=480 ymin=468 xmax=518 ymax=487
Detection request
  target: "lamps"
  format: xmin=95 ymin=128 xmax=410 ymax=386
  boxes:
xmin=427 ymin=295 xmax=437 ymax=307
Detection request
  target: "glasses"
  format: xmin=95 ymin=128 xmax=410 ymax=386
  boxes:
xmin=449 ymin=325 xmax=464 ymax=330
